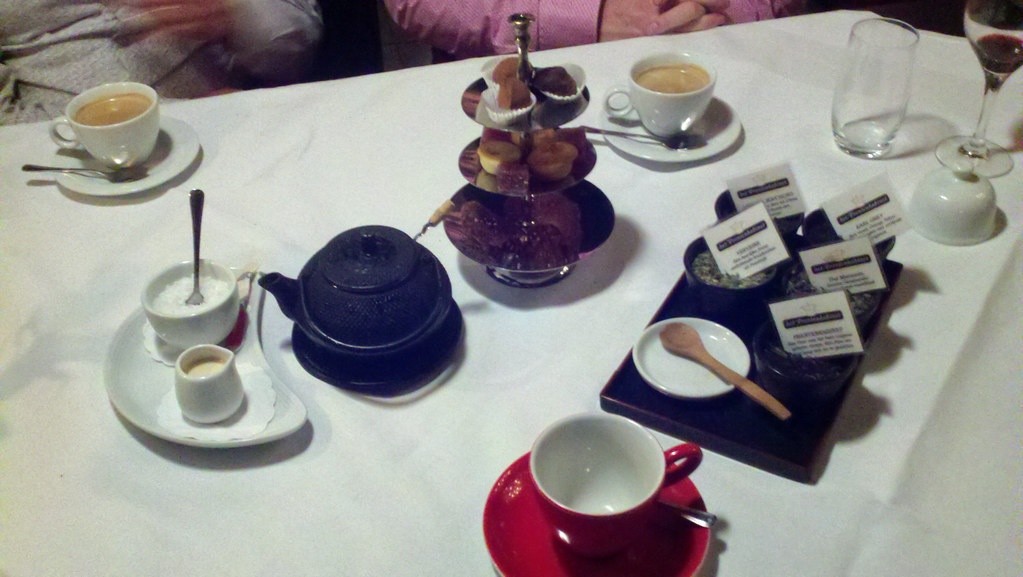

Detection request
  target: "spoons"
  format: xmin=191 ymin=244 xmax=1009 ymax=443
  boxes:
xmin=21 ymin=164 xmax=148 ymax=182
xmin=578 ymin=123 xmax=690 ymax=151
xmin=657 ymin=321 xmax=792 ymax=423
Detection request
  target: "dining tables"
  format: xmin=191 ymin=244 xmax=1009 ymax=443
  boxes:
xmin=0 ymin=9 xmax=1023 ymax=577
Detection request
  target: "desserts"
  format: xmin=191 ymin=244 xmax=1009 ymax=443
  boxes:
xmin=458 ymin=187 xmax=579 ymax=267
xmin=482 ymin=57 xmax=587 ymax=126
xmin=477 ymin=125 xmax=578 ymax=182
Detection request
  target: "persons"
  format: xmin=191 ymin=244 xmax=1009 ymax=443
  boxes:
xmin=0 ymin=0 xmax=324 ymax=124
xmin=384 ymin=0 xmax=804 ymax=62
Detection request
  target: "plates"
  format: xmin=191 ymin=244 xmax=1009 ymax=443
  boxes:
xmin=483 ymin=444 xmax=711 ymax=577
xmin=632 ymin=317 xmax=753 ymax=398
xmin=599 ymin=92 xmax=743 ymax=162
xmin=50 ymin=113 xmax=201 ymax=196
xmin=102 ymin=252 xmax=309 ymax=446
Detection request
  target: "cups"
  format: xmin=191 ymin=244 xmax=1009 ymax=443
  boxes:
xmin=175 ymin=344 xmax=245 ymax=425
xmin=49 ymin=81 xmax=161 ymax=170
xmin=600 ymin=50 xmax=718 ymax=140
xmin=529 ymin=413 xmax=702 ymax=552
xmin=140 ymin=259 xmax=242 ymax=352
xmin=832 ymin=17 xmax=920 ymax=158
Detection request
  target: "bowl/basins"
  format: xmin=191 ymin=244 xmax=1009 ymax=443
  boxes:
xmin=681 ymin=190 xmax=894 ymax=400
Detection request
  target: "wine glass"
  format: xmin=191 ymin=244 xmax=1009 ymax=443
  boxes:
xmin=931 ymin=0 xmax=1023 ymax=180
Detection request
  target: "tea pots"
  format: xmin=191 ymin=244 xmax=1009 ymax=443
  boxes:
xmin=257 ymin=223 xmax=452 ymax=356
